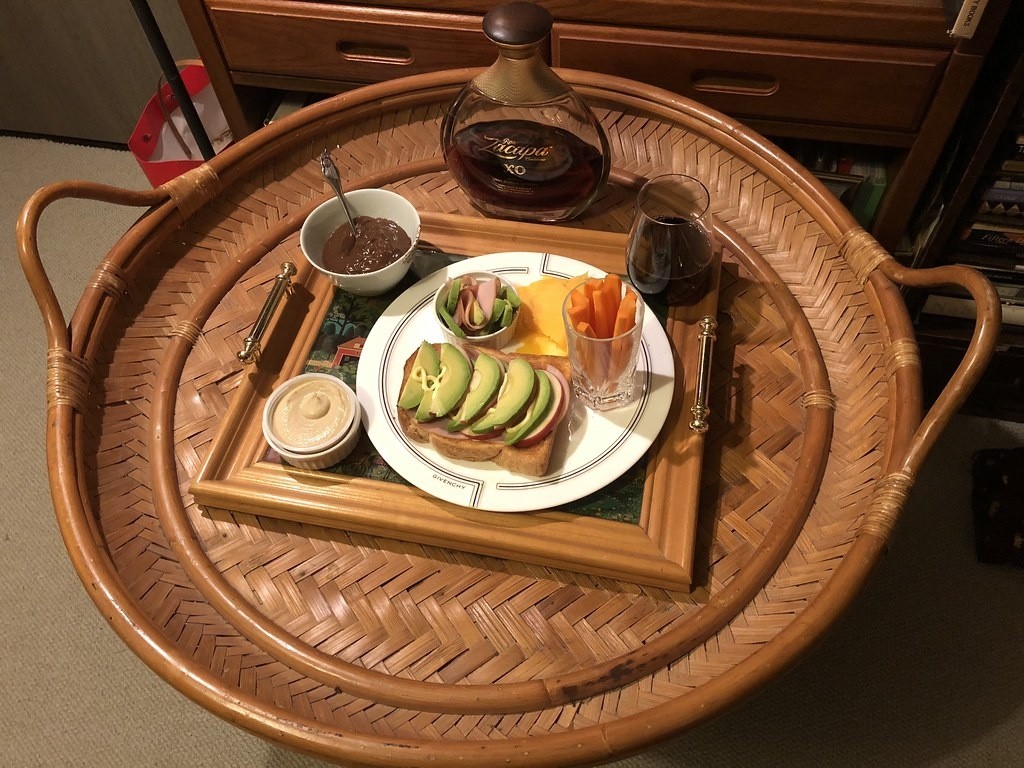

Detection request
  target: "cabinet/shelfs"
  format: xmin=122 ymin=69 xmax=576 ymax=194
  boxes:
xmin=175 ymin=0 xmax=1012 ymax=418
xmin=898 ymin=0 xmax=1024 ymax=397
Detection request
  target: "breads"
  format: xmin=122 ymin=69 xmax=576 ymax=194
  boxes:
xmin=396 ymin=342 xmax=572 ymax=477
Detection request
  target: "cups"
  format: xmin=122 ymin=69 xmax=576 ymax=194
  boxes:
xmin=626 ymin=172 xmax=715 ymax=306
xmin=560 ymin=279 xmax=645 ymax=411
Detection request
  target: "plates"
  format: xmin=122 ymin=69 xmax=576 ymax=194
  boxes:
xmin=353 ymin=250 xmax=675 ymax=513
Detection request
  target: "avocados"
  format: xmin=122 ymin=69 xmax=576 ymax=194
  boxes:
xmin=439 ymin=278 xmax=522 ymax=339
xmin=397 ymin=340 xmax=553 ymax=447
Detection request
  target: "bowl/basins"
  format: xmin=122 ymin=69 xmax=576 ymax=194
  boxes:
xmin=432 ymin=272 xmax=521 ymax=351
xmin=299 ymin=188 xmax=422 ymax=298
xmin=262 ymin=373 xmax=363 ymax=470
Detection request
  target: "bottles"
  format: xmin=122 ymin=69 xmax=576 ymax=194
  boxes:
xmin=440 ymin=0 xmax=612 ymax=225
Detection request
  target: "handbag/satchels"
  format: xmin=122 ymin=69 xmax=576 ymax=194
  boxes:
xmin=128 ymin=57 xmax=235 ymax=189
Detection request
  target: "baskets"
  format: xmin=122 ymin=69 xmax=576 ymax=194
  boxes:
xmin=17 ymin=64 xmax=1003 ymax=768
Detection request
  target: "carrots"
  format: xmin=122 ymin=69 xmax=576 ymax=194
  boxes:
xmin=567 ymin=273 xmax=637 ymax=394
